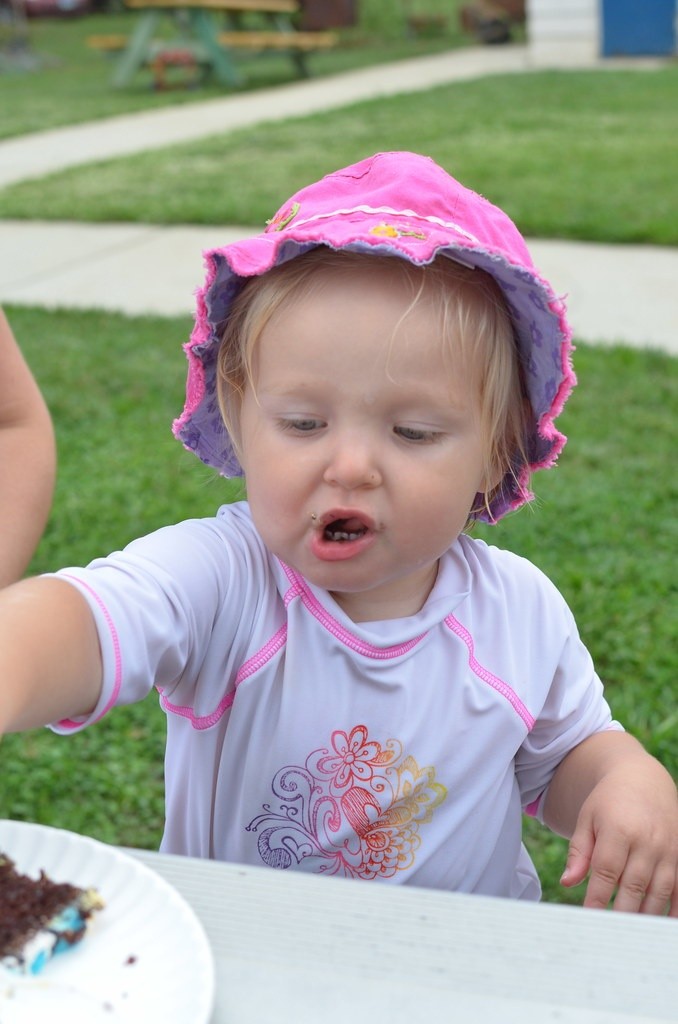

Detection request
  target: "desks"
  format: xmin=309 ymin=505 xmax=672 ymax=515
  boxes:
xmin=110 ymin=845 xmax=678 ymax=1024
xmin=111 ymin=0 xmax=312 ymax=91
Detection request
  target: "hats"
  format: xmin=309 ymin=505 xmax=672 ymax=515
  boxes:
xmin=171 ymin=150 xmax=578 ymax=527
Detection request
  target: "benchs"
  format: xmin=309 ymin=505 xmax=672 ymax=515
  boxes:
xmin=84 ymin=31 xmax=345 ymax=55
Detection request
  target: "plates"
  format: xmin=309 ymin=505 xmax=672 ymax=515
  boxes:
xmin=0 ymin=819 xmax=215 ymax=1024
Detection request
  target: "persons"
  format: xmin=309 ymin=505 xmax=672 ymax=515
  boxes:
xmin=0 ymin=149 xmax=678 ymax=917
xmin=0 ymin=309 xmax=56 ymax=589
